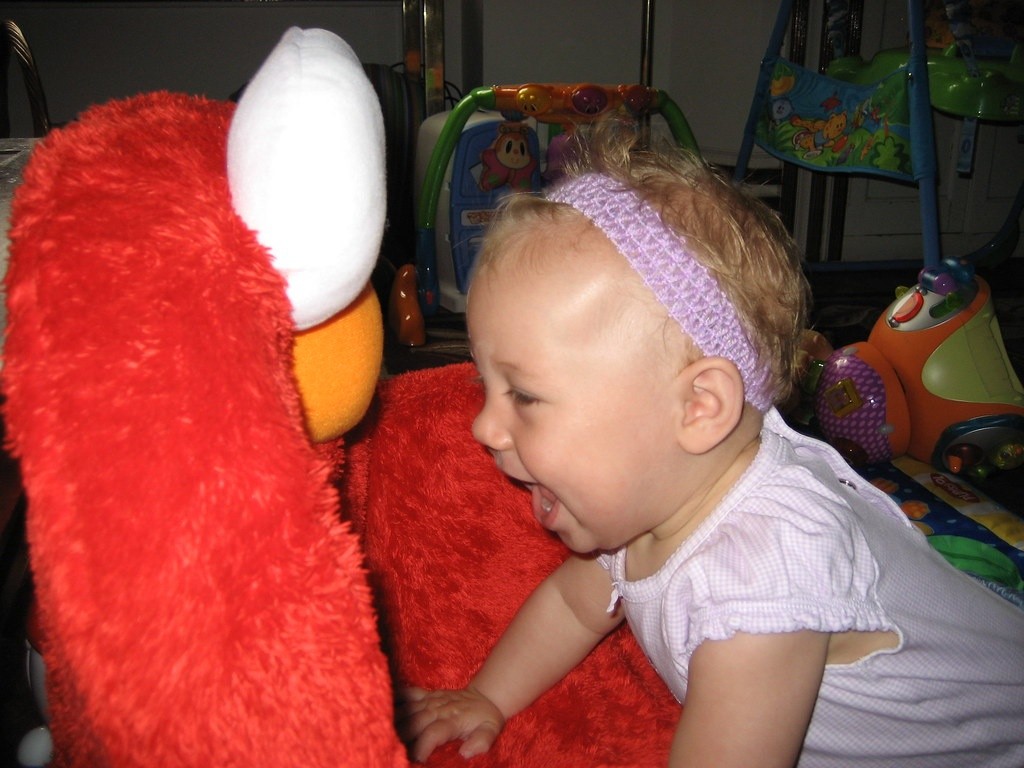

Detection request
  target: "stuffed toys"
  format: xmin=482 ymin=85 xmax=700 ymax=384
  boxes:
xmin=1 ymin=27 xmax=689 ymax=768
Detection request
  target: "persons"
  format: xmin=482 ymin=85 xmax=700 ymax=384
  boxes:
xmin=389 ymin=108 xmax=1024 ymax=768
